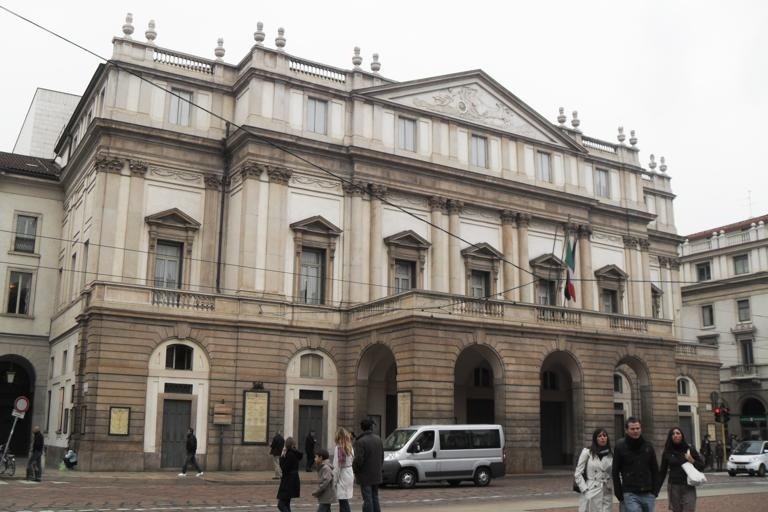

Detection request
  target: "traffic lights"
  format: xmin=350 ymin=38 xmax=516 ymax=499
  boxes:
xmin=714 ymin=408 xmax=722 ymax=423
xmin=723 ymin=407 xmax=730 ymax=422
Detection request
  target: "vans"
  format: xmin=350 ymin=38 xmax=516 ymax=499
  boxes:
xmin=379 ymin=422 xmax=508 ymax=488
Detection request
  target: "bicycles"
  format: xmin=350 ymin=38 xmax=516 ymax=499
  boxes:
xmin=0 ymin=442 xmax=16 ymax=477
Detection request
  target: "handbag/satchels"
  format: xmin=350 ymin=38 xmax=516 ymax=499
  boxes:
xmin=572 ymin=451 xmax=590 ymax=493
xmin=681 ymin=448 xmax=707 ymax=486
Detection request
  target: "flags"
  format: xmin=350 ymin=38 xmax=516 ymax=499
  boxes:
xmin=564 ymin=229 xmax=576 ymax=302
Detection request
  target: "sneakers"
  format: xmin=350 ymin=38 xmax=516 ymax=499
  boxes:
xmin=177 ymin=472 xmax=205 ymax=477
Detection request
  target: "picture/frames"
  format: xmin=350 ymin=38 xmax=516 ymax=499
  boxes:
xmin=241 ymin=389 xmax=271 ymax=446
xmin=108 ymin=406 xmax=131 ymax=436
xmin=396 ymin=390 xmax=413 ymax=428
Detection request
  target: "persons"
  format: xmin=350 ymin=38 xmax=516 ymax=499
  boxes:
xmin=270 ymin=430 xmax=285 ymax=479
xmin=575 ymin=428 xmax=614 ymax=512
xmin=331 ymin=428 xmax=356 ymax=511
xmin=25 ymin=425 xmax=44 ymax=481
xmin=613 ymin=416 xmax=659 ymax=512
xmin=277 ymin=437 xmax=303 ymax=512
xmin=352 ymin=420 xmax=384 ymax=511
xmin=311 ymin=448 xmax=337 ymax=512
xmin=656 ymin=427 xmax=702 ymax=512
xmin=177 ymin=427 xmax=204 ymax=477
xmin=701 ymin=432 xmax=748 ymax=471
xmin=304 ymin=430 xmax=317 ymax=472
xmin=64 ymin=447 xmax=78 ymax=470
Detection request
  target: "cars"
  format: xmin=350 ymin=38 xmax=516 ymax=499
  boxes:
xmin=726 ymin=439 xmax=768 ymax=477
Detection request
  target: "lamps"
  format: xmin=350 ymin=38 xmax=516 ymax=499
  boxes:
xmin=6 ymin=360 xmax=16 ymax=383
